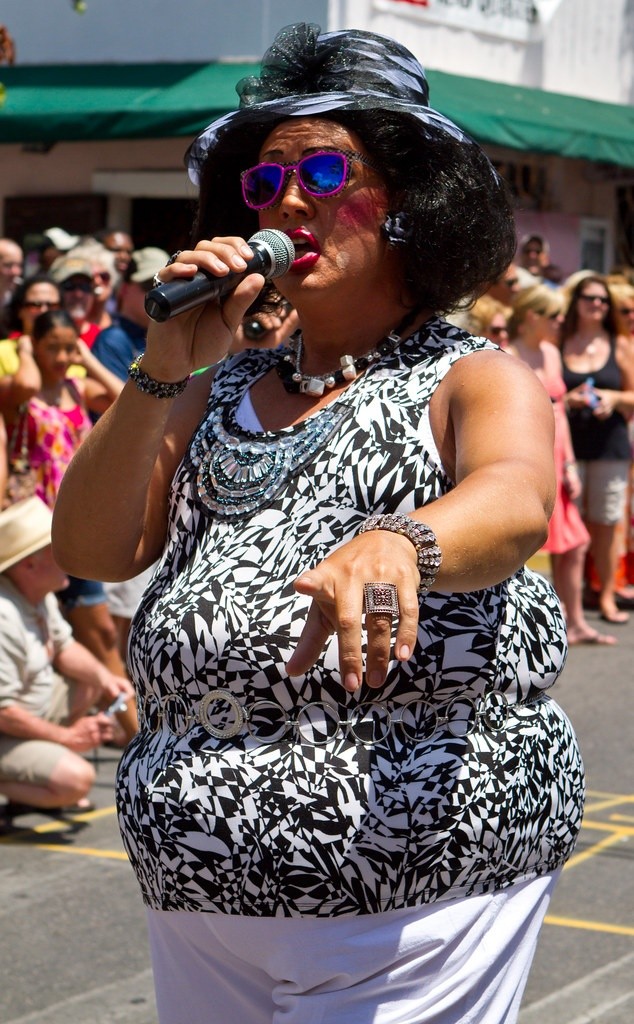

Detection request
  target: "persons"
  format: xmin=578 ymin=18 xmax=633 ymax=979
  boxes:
xmin=1 ymin=225 xmax=306 ymax=815
xmin=467 ymin=234 xmax=634 ymax=644
xmin=51 ymin=25 xmax=586 ymax=1021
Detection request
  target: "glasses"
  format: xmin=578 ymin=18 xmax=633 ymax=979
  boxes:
xmin=581 ymin=295 xmax=606 ymax=303
xmin=618 ymin=307 xmax=634 ymax=314
xmin=490 ymin=324 xmax=508 ymax=336
xmin=524 ymin=248 xmax=539 ymax=254
xmin=535 ymin=309 xmax=559 ymax=319
xmin=134 ymin=281 xmax=155 ymax=292
xmin=98 ymin=271 xmax=109 ymax=281
xmin=64 ymin=282 xmax=90 ymax=293
xmin=240 ymin=149 xmax=384 ymax=209
xmin=25 ymin=299 xmax=60 ymax=311
xmin=504 ymin=279 xmax=518 ymax=286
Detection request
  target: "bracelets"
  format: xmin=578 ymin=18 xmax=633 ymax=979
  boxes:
xmin=128 ymin=354 xmax=191 ymax=398
xmin=359 ymin=514 xmax=441 ymax=603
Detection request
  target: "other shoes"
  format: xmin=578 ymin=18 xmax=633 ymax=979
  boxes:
xmin=52 ymin=798 xmax=94 ymax=813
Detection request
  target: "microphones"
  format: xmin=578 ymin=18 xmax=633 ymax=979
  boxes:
xmin=143 ymin=228 xmax=295 ymax=322
xmin=243 ymin=296 xmax=290 ymax=341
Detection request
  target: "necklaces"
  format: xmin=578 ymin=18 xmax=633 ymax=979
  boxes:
xmin=277 ymin=303 xmax=431 ymax=397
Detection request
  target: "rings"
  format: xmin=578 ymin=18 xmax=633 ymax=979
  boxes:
xmin=362 ymin=583 xmax=400 ymax=617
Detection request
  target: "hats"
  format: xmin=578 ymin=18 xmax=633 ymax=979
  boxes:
xmin=123 ymin=247 xmax=172 ymax=282
xmin=50 ymin=257 xmax=95 ymax=284
xmin=0 ymin=496 xmax=53 ymax=574
xmin=37 ymin=228 xmax=80 ymax=251
xmin=184 ymin=22 xmax=498 ymax=193
